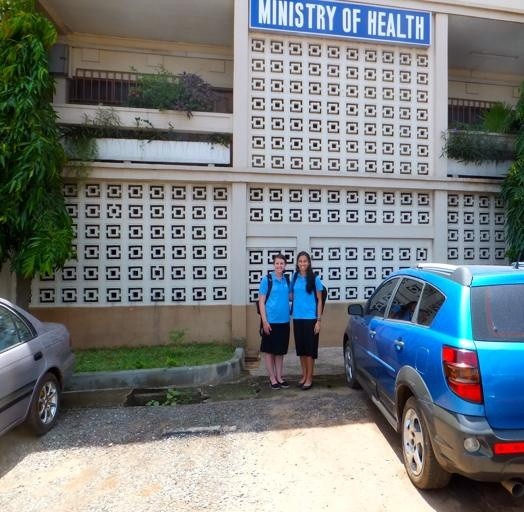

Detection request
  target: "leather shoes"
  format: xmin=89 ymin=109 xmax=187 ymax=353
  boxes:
xmin=270 ymin=378 xmax=290 ymax=389
xmin=297 ymin=378 xmax=313 ymax=390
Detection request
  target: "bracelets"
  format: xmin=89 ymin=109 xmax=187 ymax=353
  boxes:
xmin=316 ymin=316 xmax=321 ymax=319
xmin=317 ymin=320 xmax=321 ymax=321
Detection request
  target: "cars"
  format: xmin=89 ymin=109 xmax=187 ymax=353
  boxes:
xmin=0 ymin=291 xmax=73 ymax=440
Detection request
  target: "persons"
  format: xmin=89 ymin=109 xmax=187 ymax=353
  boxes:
xmin=257 ymin=253 xmax=292 ymax=391
xmin=287 ymin=249 xmax=324 ymax=391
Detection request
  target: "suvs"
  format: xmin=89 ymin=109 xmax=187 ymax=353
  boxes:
xmin=345 ymin=261 xmax=523 ymax=497
